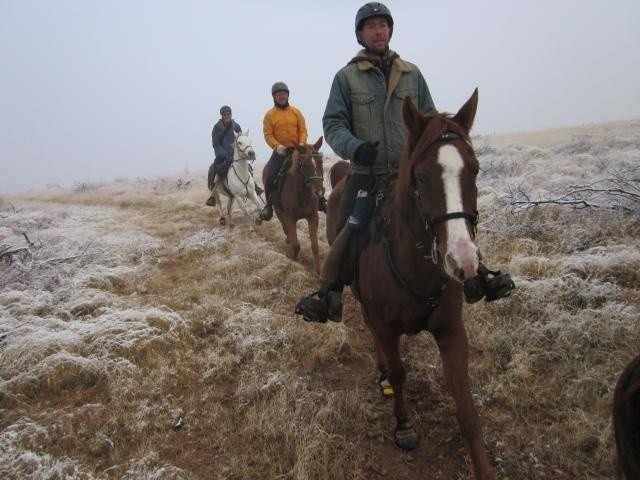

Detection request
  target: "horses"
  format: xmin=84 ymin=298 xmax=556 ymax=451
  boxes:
xmin=326 ymin=86 xmax=497 ymax=480
xmin=262 ymin=135 xmax=325 ymax=277
xmin=211 ymin=129 xmax=263 ymax=230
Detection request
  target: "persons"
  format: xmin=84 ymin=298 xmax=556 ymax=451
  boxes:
xmin=296 ymin=2 xmax=511 ymax=323
xmin=258 ymin=81 xmax=328 ymax=220
xmin=204 ymin=105 xmax=264 ymax=206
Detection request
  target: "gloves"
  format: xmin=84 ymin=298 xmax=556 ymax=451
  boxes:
xmin=354 ymin=141 xmax=380 ymax=166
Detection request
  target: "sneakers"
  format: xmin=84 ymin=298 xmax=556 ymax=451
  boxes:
xmin=205 ymin=197 xmax=216 ymax=206
xmin=325 ymin=292 xmax=344 ymax=323
xmin=320 ymin=198 xmax=327 ymax=213
xmin=253 ymin=182 xmax=264 ymax=195
xmin=259 ymin=205 xmax=274 ymax=221
xmin=463 ymin=270 xmax=516 ymax=304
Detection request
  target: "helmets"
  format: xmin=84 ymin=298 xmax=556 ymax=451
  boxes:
xmin=220 ymin=106 xmax=232 ymax=114
xmin=272 ymin=82 xmax=290 ymax=95
xmin=355 ymin=1 xmax=393 ymax=33
